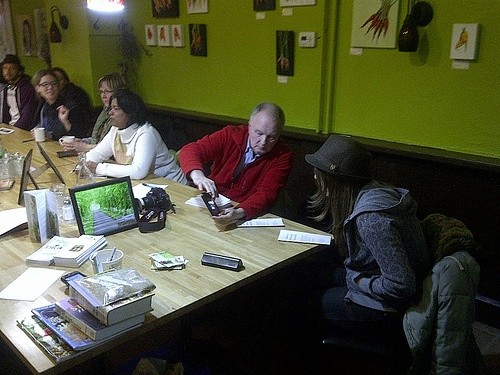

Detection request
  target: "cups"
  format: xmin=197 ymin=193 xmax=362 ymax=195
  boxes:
xmin=59 ymin=136 xmax=75 ymax=150
xmin=89 ymin=249 xmax=124 ymax=274
xmin=33 ymin=128 xmax=46 ymax=142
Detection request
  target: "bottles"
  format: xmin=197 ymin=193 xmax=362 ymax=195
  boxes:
xmin=52 ymin=183 xmax=75 ymax=222
xmin=0 ymin=151 xmax=29 ymax=178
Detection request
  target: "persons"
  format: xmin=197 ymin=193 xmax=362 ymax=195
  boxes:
xmin=82 ymin=89 xmax=188 ymax=185
xmin=58 ymin=72 xmax=126 ymax=151
xmin=177 ymin=101 xmax=293 ymax=232
xmin=305 ymin=133 xmax=426 ymax=331
xmin=0 ymin=53 xmax=95 ymax=141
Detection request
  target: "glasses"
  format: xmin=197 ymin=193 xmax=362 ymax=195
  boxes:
xmin=38 ymin=80 xmax=60 ymax=87
xmin=98 ymin=90 xmax=113 ymax=95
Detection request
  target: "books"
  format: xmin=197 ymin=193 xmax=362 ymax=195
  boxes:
xmin=16 ymin=268 xmax=155 ymax=365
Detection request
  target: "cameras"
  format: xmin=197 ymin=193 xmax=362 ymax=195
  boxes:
xmin=143 ymin=188 xmax=171 ymax=211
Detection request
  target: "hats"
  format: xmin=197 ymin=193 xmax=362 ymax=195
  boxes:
xmin=0 ymin=54 xmax=25 ymax=71
xmin=305 ymin=135 xmax=370 ymax=180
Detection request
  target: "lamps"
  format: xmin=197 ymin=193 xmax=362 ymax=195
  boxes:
xmin=87 ymin=0 xmax=125 ymax=12
xmin=398 ymin=2 xmax=433 ymax=53
xmin=49 ymin=5 xmax=68 ymax=44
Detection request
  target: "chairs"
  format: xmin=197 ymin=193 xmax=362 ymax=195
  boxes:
xmin=322 ymin=250 xmax=486 ymax=375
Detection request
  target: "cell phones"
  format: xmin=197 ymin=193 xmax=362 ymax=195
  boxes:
xmin=202 ymin=252 xmax=243 ymax=270
xmin=58 ymin=149 xmax=78 ymax=157
xmin=61 ymin=271 xmax=86 ymax=285
xmin=201 ymin=193 xmax=221 ymax=216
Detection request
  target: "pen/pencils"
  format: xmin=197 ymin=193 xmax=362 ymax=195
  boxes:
xmin=141 ymin=180 xmax=154 ymax=189
xmin=22 ymin=138 xmax=36 ymax=143
xmin=110 ymin=245 xmax=117 ymax=261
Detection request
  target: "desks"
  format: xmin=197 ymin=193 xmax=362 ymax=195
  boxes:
xmin=0 ymin=122 xmax=335 ymax=375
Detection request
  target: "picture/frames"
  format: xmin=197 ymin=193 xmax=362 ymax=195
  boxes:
xmin=69 ymin=175 xmax=137 ymax=237
